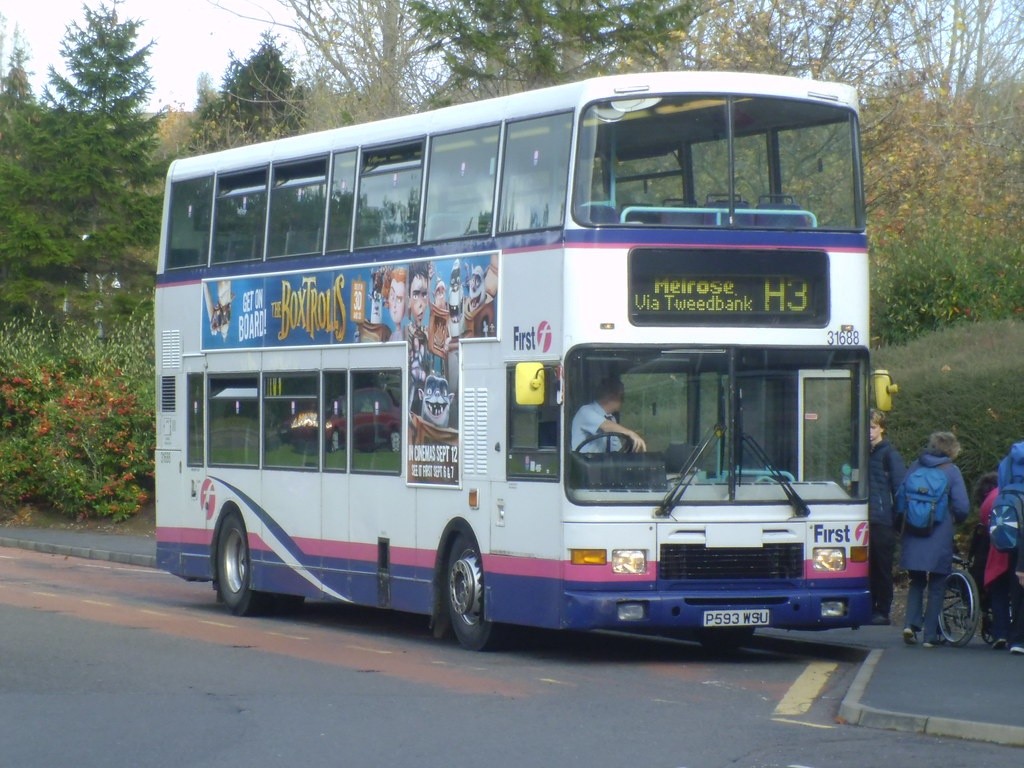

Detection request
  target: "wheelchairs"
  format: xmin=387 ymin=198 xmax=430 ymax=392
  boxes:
xmin=937 ymin=518 xmax=1009 ymax=654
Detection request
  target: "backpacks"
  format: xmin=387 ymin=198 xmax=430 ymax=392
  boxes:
xmin=894 ymin=466 xmax=949 ymax=536
xmin=989 ymin=455 xmax=1024 ymax=554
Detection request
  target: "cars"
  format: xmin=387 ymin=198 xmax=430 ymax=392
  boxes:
xmin=271 ymin=387 xmax=404 ymax=456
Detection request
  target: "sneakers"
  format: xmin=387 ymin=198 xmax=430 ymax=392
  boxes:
xmin=1011 ymin=640 xmax=1023 ymax=653
xmin=993 ymin=631 xmax=1007 ymax=651
xmin=902 ymin=623 xmax=918 ymax=644
xmin=922 ymin=639 xmax=940 ymax=647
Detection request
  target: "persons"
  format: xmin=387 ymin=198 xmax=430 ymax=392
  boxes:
xmin=991 ymin=438 xmax=1024 ymax=654
xmin=859 ymin=409 xmax=903 ymax=625
xmin=570 ymin=378 xmax=648 ymax=452
xmin=892 ymin=432 xmax=968 ymax=645
xmin=974 ymin=470 xmax=1014 ymax=645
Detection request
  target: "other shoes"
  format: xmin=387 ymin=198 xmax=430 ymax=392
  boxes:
xmin=868 ymin=614 xmax=889 ymax=625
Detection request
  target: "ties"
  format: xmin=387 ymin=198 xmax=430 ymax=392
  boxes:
xmin=604 ymin=415 xmax=614 ymax=452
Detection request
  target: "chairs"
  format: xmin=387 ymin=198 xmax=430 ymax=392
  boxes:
xmin=167 ymin=185 xmax=811 ymax=267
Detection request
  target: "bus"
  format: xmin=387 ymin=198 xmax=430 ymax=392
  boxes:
xmin=149 ymin=66 xmax=900 ymax=652
